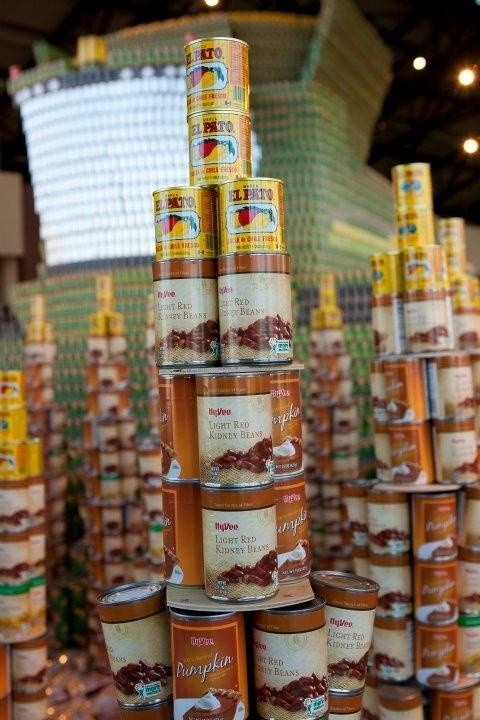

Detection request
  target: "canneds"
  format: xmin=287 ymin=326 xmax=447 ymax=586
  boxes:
xmin=0 ymin=163 xmax=480 ymax=719
xmin=188 ymin=110 xmax=252 ymax=188
xmin=184 ymin=38 xmax=250 ymax=118
